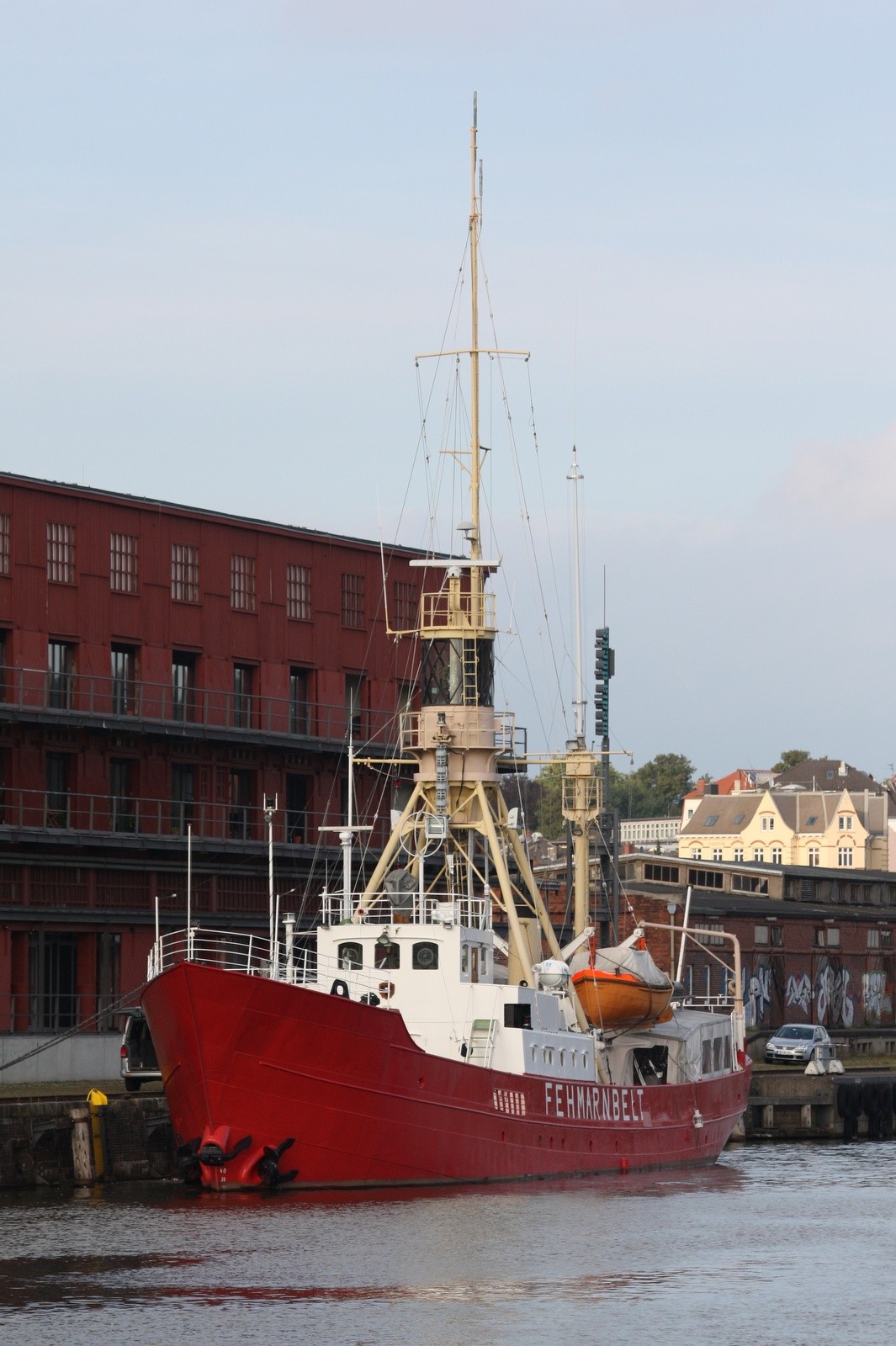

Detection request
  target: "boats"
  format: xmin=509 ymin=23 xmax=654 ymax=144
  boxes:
xmin=568 ymin=969 xmax=673 ymax=1030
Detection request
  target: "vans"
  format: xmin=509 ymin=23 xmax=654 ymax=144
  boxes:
xmin=110 ymin=1004 xmax=163 ymax=1092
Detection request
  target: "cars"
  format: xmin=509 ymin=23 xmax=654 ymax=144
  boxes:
xmin=764 ymin=1023 xmax=832 ymax=1065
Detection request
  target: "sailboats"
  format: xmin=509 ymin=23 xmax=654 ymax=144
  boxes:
xmin=139 ymin=89 xmax=754 ymax=1202
